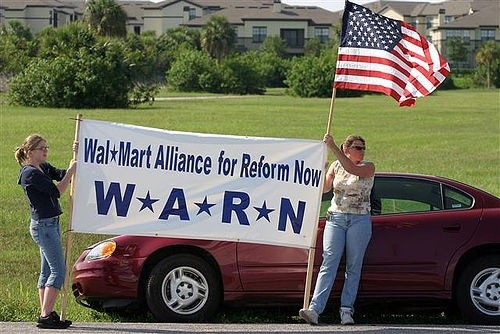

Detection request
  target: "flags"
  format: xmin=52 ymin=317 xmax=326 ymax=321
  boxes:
xmin=334 ymin=1 xmax=451 ymax=107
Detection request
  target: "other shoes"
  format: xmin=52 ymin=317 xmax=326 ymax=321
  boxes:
xmin=36 ymin=310 xmax=73 ymax=330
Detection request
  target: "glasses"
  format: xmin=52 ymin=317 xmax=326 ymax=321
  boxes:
xmin=32 ymin=146 xmax=50 ymax=151
xmin=349 ymin=146 xmax=366 ymax=151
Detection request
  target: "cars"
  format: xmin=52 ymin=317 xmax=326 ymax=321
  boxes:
xmin=74 ymin=172 xmax=500 ymax=326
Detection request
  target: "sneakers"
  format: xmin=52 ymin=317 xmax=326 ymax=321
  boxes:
xmin=299 ymin=308 xmax=319 ymax=325
xmin=339 ymin=309 xmax=354 ymax=325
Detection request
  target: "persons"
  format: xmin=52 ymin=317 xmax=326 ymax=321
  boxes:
xmin=299 ymin=134 xmax=375 ymax=325
xmin=15 ymin=133 xmax=77 ymax=329
